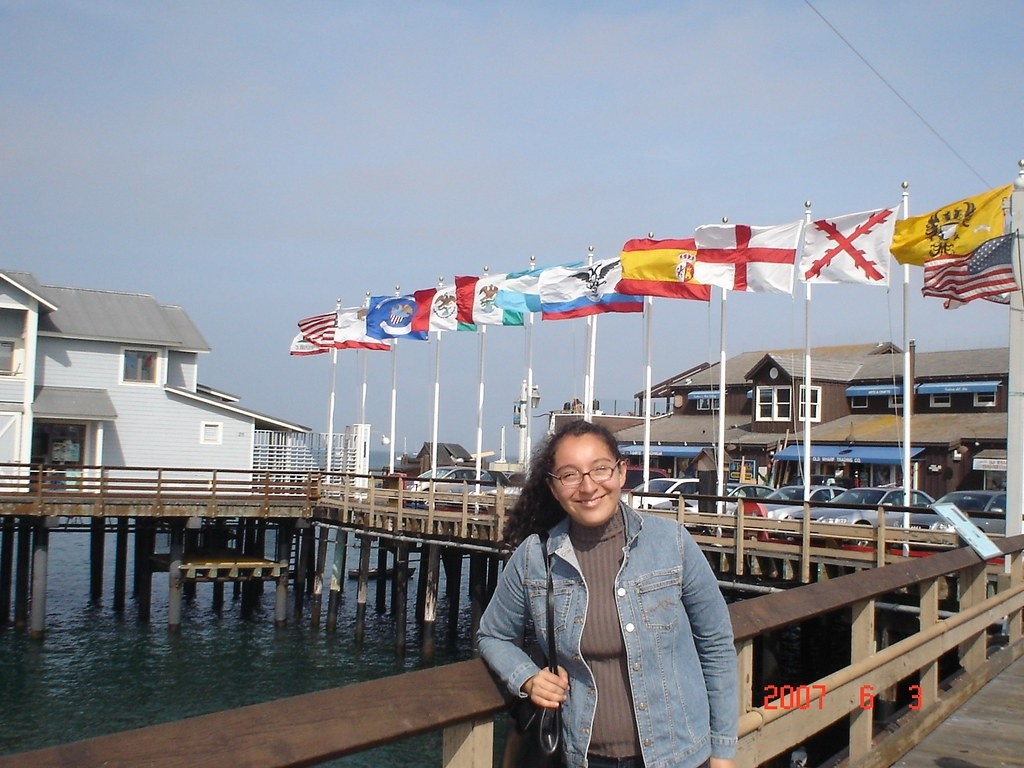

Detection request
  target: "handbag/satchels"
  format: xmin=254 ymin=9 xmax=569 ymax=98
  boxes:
xmin=507 ymin=531 xmax=562 ymax=768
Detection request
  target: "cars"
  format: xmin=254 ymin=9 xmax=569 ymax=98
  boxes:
xmin=622 ymin=477 xmax=739 ymax=512
xmin=893 ymin=490 xmax=1007 ymax=551
xmin=405 ymin=466 xmax=497 ymax=504
xmin=653 ymin=483 xmax=788 ymax=519
xmin=760 ymin=483 xmax=865 ymax=525
xmin=788 ymin=487 xmax=949 ymax=529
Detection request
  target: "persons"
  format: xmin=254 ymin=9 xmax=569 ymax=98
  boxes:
xmin=656 ymin=412 xmax=660 ymax=416
xmin=477 ymin=417 xmax=740 ymax=768
xmin=702 ymin=399 xmax=708 ymax=408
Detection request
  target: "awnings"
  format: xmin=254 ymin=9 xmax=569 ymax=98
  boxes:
xmin=746 ymin=388 xmax=772 ymax=398
xmin=617 ymin=444 xmax=714 ymax=456
xmin=845 ymin=385 xmax=903 ymax=396
xmin=773 ymin=446 xmax=925 ymax=467
xmin=918 ymin=381 xmax=1003 ymax=395
xmin=973 ymin=447 xmax=1007 ymax=471
xmin=688 ymin=390 xmax=720 ymax=399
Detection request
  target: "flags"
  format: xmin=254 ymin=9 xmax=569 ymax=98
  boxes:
xmin=290 ymin=331 xmax=332 ymax=356
xmin=695 ymin=220 xmax=804 ymax=298
xmin=615 ymin=237 xmax=710 ymax=303
xmin=494 ymin=261 xmax=586 ymax=313
xmin=891 ymin=184 xmax=1012 ymax=268
xmin=798 ymin=202 xmax=903 ymax=286
xmin=299 ymin=311 xmax=337 ymax=347
xmin=412 ymin=285 xmax=479 ymax=332
xmin=334 ymin=305 xmax=391 ymax=351
xmin=539 ymin=256 xmax=646 ymax=320
xmin=365 ymin=294 xmax=430 ymax=343
xmin=921 ymin=236 xmax=1020 ymax=310
xmin=456 ymin=270 xmax=532 ymax=326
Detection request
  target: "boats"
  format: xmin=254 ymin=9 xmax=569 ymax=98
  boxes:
xmin=348 ymin=567 xmax=416 ymax=580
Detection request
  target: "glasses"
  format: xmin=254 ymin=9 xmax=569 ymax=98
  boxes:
xmin=547 ymin=458 xmax=621 ymax=486
